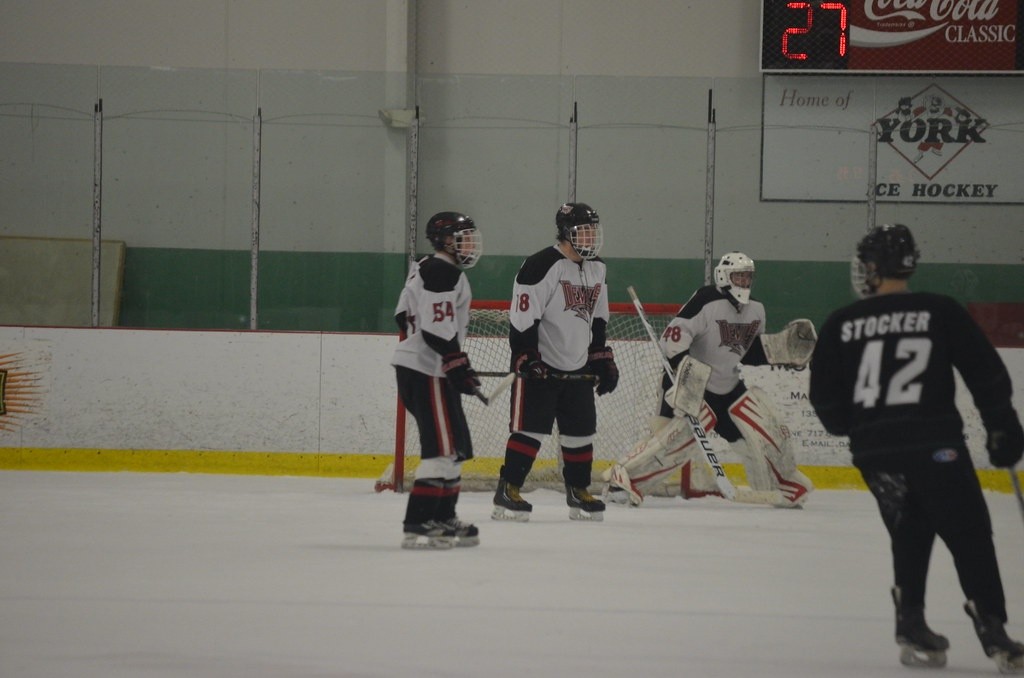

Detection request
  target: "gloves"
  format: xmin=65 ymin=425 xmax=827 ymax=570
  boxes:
xmin=588 ymin=346 xmax=619 ymax=396
xmin=441 ymin=352 xmax=481 ymax=396
xmin=514 ymin=349 xmax=551 ymax=385
xmin=982 ymin=409 xmax=1024 ymax=468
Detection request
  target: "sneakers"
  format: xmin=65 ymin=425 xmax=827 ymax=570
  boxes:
xmin=434 ymin=517 xmax=480 ymax=547
xmin=891 ymin=587 xmax=949 ymax=668
xmin=401 ymin=521 xmax=458 ymax=550
xmin=562 ymin=466 xmax=606 ymax=521
xmin=964 ymin=600 xmax=1024 ymax=673
xmin=491 ymin=465 xmax=532 ymax=522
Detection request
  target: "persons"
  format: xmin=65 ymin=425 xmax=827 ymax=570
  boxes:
xmin=807 ymin=224 xmax=1023 ymax=678
xmin=601 ymin=249 xmax=817 ymax=505
xmin=388 ymin=212 xmax=482 ymax=551
xmin=490 ymin=201 xmax=620 ymax=522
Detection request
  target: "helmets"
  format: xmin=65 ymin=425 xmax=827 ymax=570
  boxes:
xmin=426 ymin=211 xmax=482 ymax=269
xmin=851 ymin=225 xmax=917 ymax=298
xmin=556 ymin=203 xmax=603 ymax=260
xmin=714 ymin=252 xmax=755 ymax=304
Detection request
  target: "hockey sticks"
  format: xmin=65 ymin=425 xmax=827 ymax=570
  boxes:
xmin=478 ymin=371 xmax=601 ymax=382
xmin=1009 ymin=467 xmax=1023 ymax=521
xmin=475 ymin=370 xmax=516 ymax=408
xmin=626 ymin=286 xmax=783 ymax=507
